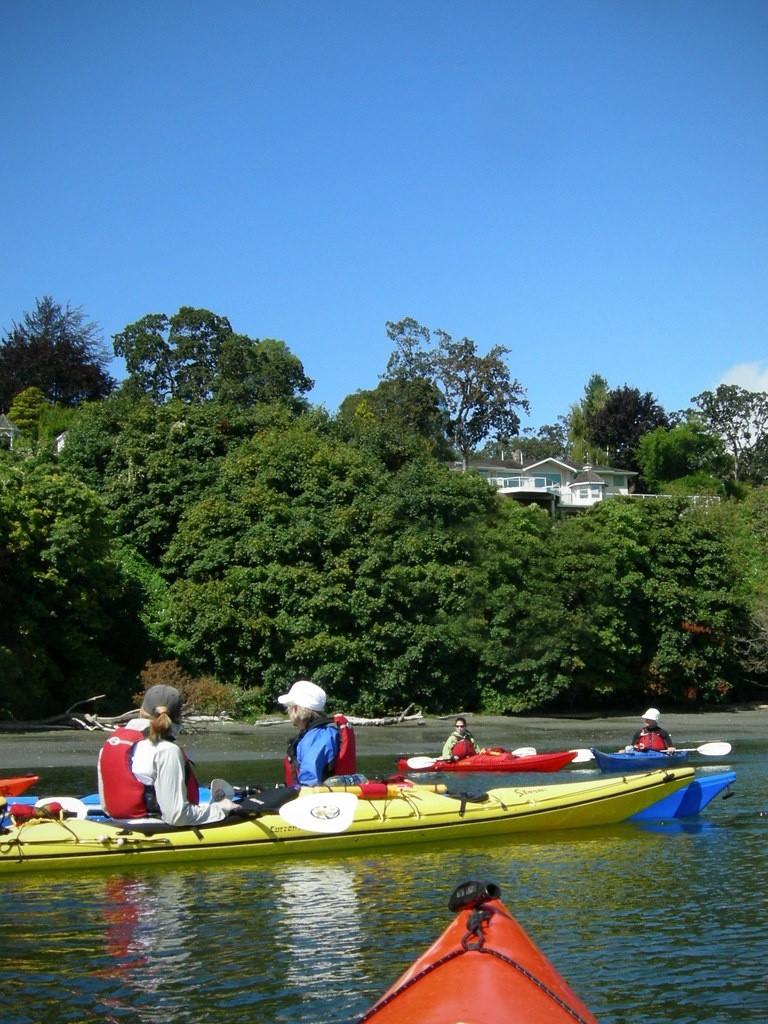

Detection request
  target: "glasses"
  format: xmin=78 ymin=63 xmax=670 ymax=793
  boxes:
xmin=455 ymin=725 xmax=465 ymax=728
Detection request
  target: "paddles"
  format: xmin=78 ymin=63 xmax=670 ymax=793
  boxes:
xmin=35 ymin=791 xmax=358 ymax=833
xmin=568 ymin=741 xmax=732 ymax=763
xmin=407 ymin=747 xmax=537 ymax=770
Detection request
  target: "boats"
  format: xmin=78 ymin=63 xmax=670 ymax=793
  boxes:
xmin=0 ymin=767 xmax=737 ymax=878
xmin=589 ymin=747 xmax=699 ymax=774
xmin=354 ymin=882 xmax=601 ymax=1024
xmin=398 ymin=751 xmax=578 ymax=771
xmin=1 ymin=776 xmax=40 ymax=797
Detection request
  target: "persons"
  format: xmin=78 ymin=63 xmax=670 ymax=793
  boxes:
xmin=277 ymin=680 xmax=341 ymax=787
xmin=442 ymin=717 xmax=482 ymax=763
xmin=632 ymin=708 xmax=676 ymax=756
xmin=97 ymin=685 xmax=243 ymax=826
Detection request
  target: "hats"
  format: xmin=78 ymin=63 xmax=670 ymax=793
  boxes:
xmin=142 ymin=685 xmax=182 ymax=718
xmin=641 ymin=708 xmax=660 ymax=724
xmin=278 ymin=680 xmax=327 ymax=711
